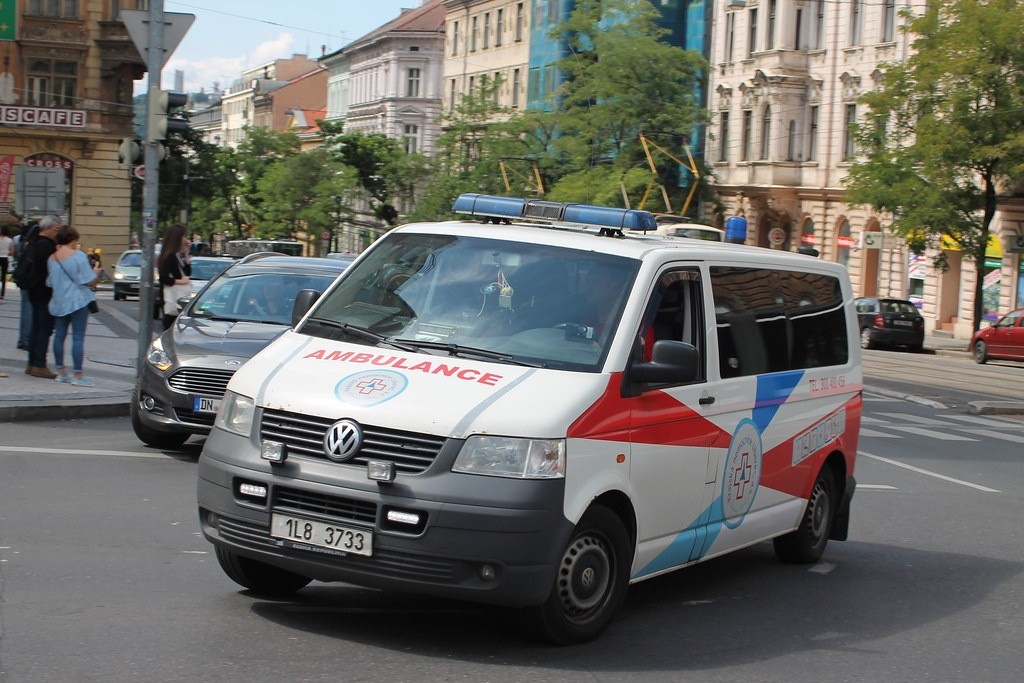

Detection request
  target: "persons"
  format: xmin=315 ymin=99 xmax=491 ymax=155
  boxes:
xmin=548 ymin=257 xmax=655 ymax=364
xmin=0 ymin=214 xmax=104 ymax=387
xmin=158 ymin=224 xmax=192 ymax=331
xmin=248 ymin=277 xmax=292 ymax=317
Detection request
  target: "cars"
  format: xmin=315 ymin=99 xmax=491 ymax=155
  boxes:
xmin=969 ymin=307 xmax=1024 ymax=364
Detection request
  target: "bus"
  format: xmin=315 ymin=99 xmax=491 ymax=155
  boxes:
xmin=227 ymin=236 xmax=304 ymax=260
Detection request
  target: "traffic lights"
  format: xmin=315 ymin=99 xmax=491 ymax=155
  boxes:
xmin=159 ymin=90 xmax=189 ymax=141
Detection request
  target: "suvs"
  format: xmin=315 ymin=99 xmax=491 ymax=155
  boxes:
xmin=131 ymin=251 xmax=430 ymax=450
xmin=855 ymin=296 xmax=925 ymax=353
xmin=160 ymin=257 xmax=236 ymax=330
xmin=111 ymin=250 xmax=159 ymax=301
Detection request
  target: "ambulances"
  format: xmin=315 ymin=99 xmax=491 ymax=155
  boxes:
xmin=197 ymin=190 xmax=863 ymax=648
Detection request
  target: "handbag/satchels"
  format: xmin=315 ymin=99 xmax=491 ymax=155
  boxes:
xmin=162 ymin=283 xmax=199 ymax=315
xmin=88 ymin=301 xmax=99 ymax=313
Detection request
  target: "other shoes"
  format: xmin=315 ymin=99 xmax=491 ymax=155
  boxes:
xmin=54 ymin=375 xmax=72 ymax=383
xmin=25 ymin=365 xmax=35 ymax=374
xmin=31 ymin=367 xmax=56 ymax=378
xmin=18 ymin=344 xmax=29 ymax=351
xmin=0 ymin=371 xmax=9 ymax=377
xmin=73 ymin=377 xmax=95 ymax=387
